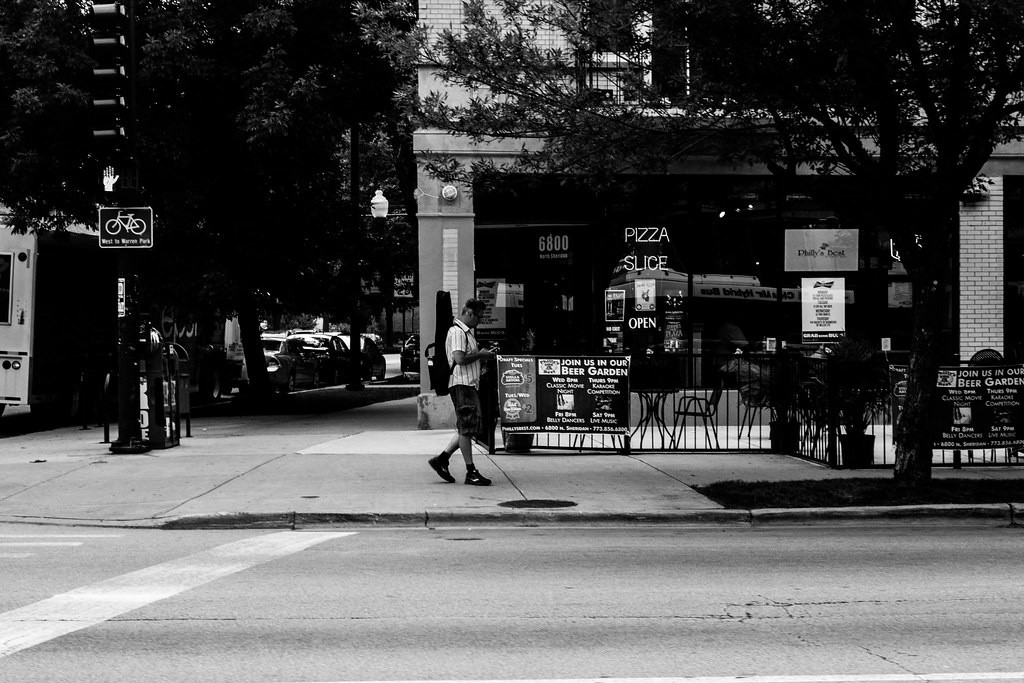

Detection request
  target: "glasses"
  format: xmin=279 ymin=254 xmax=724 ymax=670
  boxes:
xmin=473 ymin=311 xmax=483 ymax=320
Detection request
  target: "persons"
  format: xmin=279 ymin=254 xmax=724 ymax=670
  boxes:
xmin=426 ymin=298 xmax=503 ymax=486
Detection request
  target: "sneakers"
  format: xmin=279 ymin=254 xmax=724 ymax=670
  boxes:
xmin=427 ymin=456 xmax=456 ymax=483
xmin=464 ymin=469 xmax=492 ymax=486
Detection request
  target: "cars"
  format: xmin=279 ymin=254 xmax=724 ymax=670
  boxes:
xmin=218 ymin=328 xmax=421 ymax=394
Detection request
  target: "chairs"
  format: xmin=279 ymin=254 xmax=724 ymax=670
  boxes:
xmin=667 ymin=375 xmax=724 ymax=452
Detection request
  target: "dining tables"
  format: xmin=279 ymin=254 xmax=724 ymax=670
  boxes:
xmin=630 ymin=386 xmax=681 ymax=451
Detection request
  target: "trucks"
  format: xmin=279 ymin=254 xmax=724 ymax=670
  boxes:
xmin=0 ymin=227 xmax=119 ymax=423
